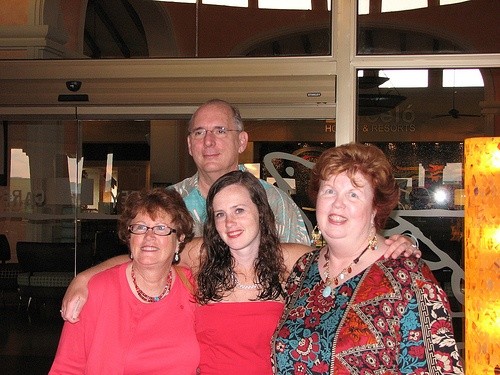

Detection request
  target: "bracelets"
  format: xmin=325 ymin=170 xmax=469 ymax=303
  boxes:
xmin=402 ymin=233 xmax=420 ymax=250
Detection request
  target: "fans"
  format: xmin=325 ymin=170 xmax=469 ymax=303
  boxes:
xmin=429 ymin=90 xmax=488 ymax=119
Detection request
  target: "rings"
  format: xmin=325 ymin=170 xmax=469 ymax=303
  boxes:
xmin=411 ymin=244 xmax=415 ymax=249
xmin=59 ymin=310 xmax=63 ymax=312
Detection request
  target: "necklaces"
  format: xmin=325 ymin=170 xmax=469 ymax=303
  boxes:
xmin=130 ymin=265 xmax=173 ymax=303
xmin=322 ymin=235 xmax=378 ymax=298
xmin=229 ymin=267 xmax=261 ymax=290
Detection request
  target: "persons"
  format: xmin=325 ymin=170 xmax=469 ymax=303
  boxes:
xmin=46 ymin=188 xmax=201 ymax=375
xmin=61 ymin=170 xmax=421 ymax=374
xmin=159 ymin=98 xmax=312 ymax=245
xmin=269 ymin=141 xmax=463 ymax=375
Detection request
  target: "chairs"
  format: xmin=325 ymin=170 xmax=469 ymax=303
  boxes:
xmin=0 ymin=234 xmax=82 ymax=333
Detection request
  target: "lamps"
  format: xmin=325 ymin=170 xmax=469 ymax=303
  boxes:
xmin=358 ymin=68 xmax=408 ymax=116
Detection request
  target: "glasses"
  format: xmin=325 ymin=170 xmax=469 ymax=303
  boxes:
xmin=127 ymin=224 xmax=176 ymax=236
xmin=192 ymin=127 xmax=241 ymax=140
